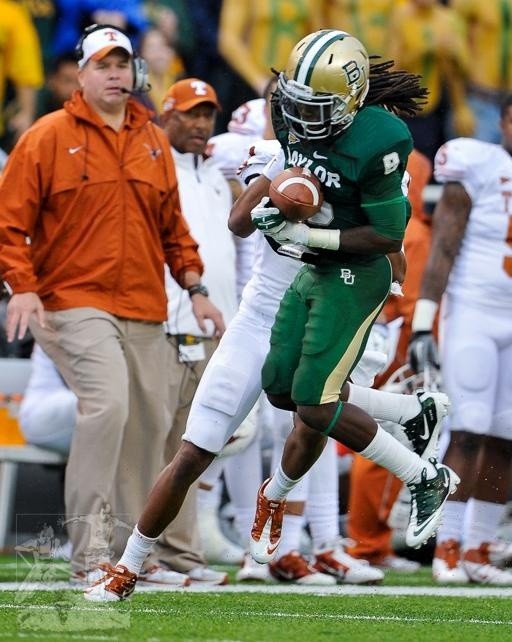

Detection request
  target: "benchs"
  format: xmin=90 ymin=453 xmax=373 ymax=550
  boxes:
xmin=1 ymin=355 xmax=67 ymax=556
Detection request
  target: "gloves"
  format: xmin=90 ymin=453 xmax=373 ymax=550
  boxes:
xmin=409 ymin=329 xmax=442 ymax=374
xmin=251 ymin=196 xmax=341 ymax=253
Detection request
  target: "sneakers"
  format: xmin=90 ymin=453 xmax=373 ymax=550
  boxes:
xmin=405 ymin=393 xmax=448 ymax=466
xmin=403 ymin=464 xmax=460 ymax=550
xmin=71 ymin=539 xmax=511 ymax=604
xmin=251 ymin=477 xmax=286 ymax=563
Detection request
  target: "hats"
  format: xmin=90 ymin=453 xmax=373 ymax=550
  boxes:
xmin=76 ymin=25 xmax=133 ymax=70
xmin=160 ymin=79 xmax=220 ymax=123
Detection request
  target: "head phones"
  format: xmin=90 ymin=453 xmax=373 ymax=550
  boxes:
xmin=74 ymin=22 xmax=152 ymax=92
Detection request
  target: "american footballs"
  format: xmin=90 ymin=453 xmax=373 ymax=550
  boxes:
xmin=269 ymin=168 xmax=322 ymax=220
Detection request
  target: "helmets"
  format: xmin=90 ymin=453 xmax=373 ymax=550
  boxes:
xmin=276 ymin=28 xmax=371 ymax=139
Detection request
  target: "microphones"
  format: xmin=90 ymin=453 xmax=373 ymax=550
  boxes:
xmin=121 ymin=87 xmax=142 ymax=96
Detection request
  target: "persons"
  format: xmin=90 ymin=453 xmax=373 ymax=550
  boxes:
xmin=0 ymin=1 xmax=512 ymax=604
